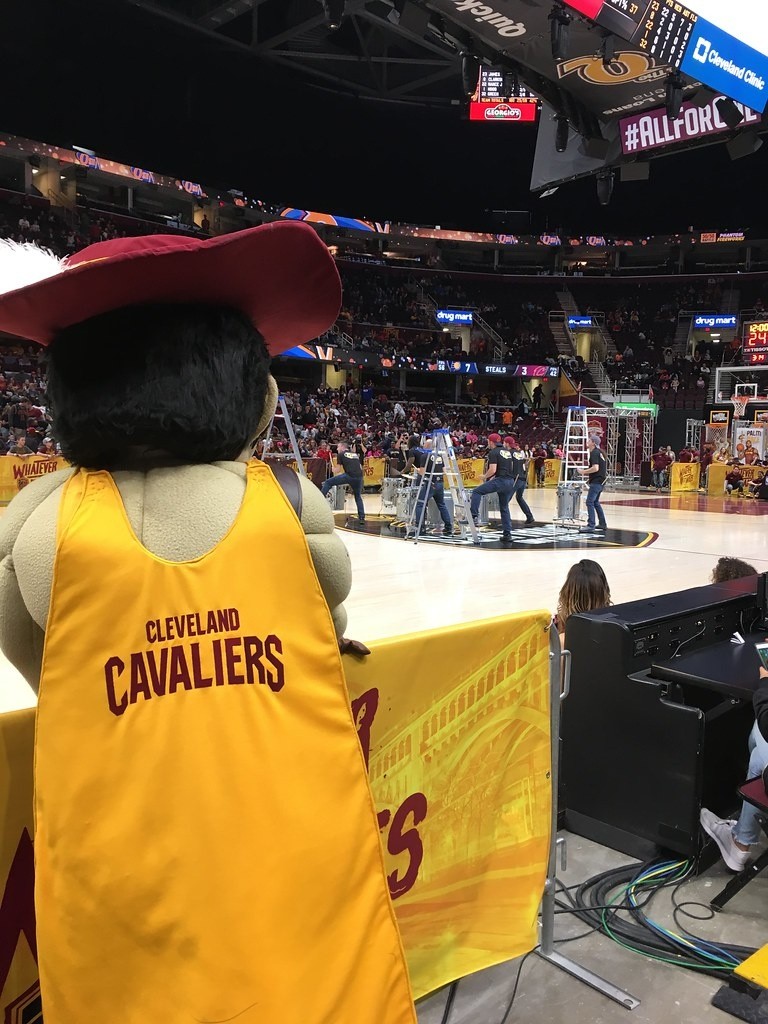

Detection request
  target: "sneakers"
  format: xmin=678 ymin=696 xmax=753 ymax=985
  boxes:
xmin=595 ymin=525 xmax=607 ymax=530
xmin=697 ymin=488 xmax=705 ymax=492
xmin=578 ymin=526 xmax=595 ymax=533
xmin=524 ymin=519 xmax=534 ymax=524
xmin=745 ymin=493 xmax=754 ymax=498
xmin=700 ymin=808 xmax=751 ymax=871
xmin=359 ymin=517 xmax=365 ymax=524
xmin=416 ymin=529 xmax=426 ymax=535
xmin=500 ymin=534 xmax=513 ymax=544
xmin=754 ymin=493 xmax=759 ymax=499
xmin=727 ymin=490 xmax=731 ymax=496
xmin=737 ymin=493 xmax=745 ymax=498
xmin=467 ymin=516 xmax=478 ymax=525
xmin=442 ymin=529 xmax=452 ymax=532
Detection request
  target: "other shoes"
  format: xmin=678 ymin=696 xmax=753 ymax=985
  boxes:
xmin=656 ymin=489 xmax=662 ymax=495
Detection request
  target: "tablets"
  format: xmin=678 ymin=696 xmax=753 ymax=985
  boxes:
xmin=754 ymin=642 xmax=768 ymax=671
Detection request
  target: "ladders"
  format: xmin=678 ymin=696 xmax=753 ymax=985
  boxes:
xmin=556 ymin=406 xmax=591 ymax=489
xmin=260 ymin=396 xmax=308 ymax=477
xmin=404 ymin=429 xmax=481 ymax=545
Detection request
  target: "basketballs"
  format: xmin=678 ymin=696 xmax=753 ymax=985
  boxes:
xmin=736 ymin=443 xmax=745 ymax=452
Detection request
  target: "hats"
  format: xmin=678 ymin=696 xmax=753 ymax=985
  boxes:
xmin=27 ymin=417 xmax=36 ymax=424
xmin=590 ymin=435 xmax=601 ymax=449
xmin=488 ymin=433 xmax=503 ymax=447
xmin=44 ymin=438 xmax=53 ymax=445
xmin=503 ymin=436 xmax=517 ymax=449
xmin=27 ymin=427 xmax=35 ymax=432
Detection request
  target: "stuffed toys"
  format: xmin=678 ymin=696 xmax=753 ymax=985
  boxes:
xmin=0 ymin=220 xmax=416 ymax=1024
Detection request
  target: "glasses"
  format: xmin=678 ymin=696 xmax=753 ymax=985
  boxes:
xmin=662 ymin=450 xmax=666 ymax=451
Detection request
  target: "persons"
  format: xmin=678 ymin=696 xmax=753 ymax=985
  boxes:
xmin=250 ymin=373 xmax=423 ymax=493
xmin=320 ymin=442 xmax=365 ymax=524
xmin=577 ymin=435 xmax=607 ymax=532
xmin=0 ymin=331 xmax=62 ymax=457
xmin=560 ymin=259 xmax=768 ymax=397
xmin=700 ymin=637 xmax=768 ymax=871
xmin=410 ymin=439 xmax=452 ymax=533
xmin=308 ymin=244 xmax=560 ymax=385
xmin=0 ymin=192 xmax=211 ymax=259
xmin=423 ymin=384 xmax=580 ymax=488
xmin=503 ymin=436 xmax=534 ymax=524
xmin=651 ymin=444 xmax=768 ymax=501
xmin=553 ymin=559 xmax=610 ymax=695
xmin=459 ymin=433 xmax=514 ymax=543
xmin=712 ymin=557 xmax=757 ymax=583
